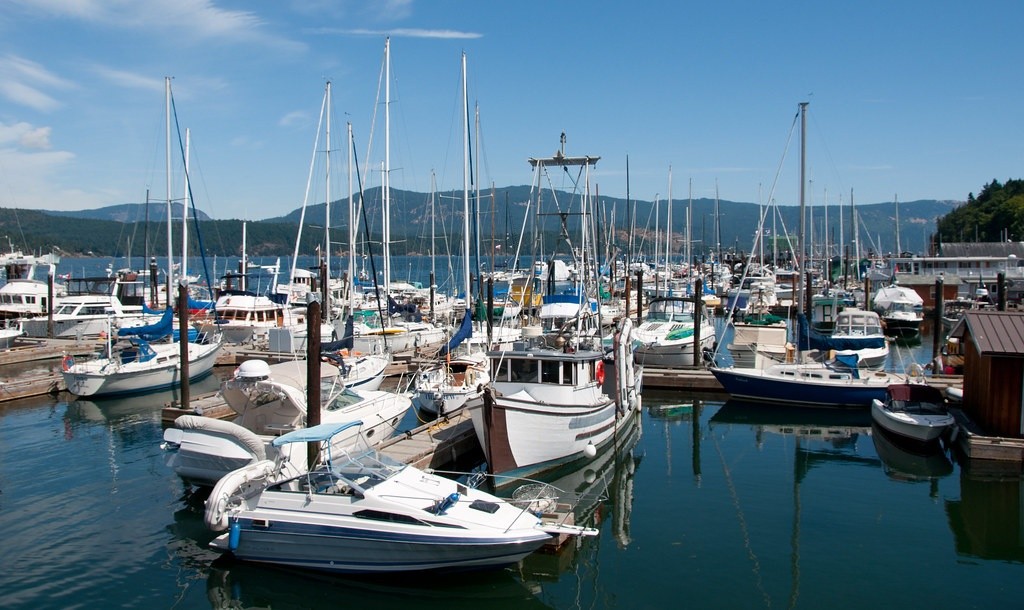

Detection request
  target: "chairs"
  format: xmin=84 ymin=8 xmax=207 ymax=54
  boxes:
xmin=431 ymin=372 xmax=466 ymax=388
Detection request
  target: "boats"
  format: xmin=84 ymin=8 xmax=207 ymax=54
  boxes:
xmin=869 ymin=344 xmax=956 ymax=444
xmin=201 ymin=421 xmax=601 ymax=571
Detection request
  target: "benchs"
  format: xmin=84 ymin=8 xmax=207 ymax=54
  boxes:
xmin=299 ymin=470 xmax=337 ymax=492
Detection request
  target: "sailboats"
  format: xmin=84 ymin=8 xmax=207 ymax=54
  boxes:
xmin=2 ymin=32 xmax=939 ymax=486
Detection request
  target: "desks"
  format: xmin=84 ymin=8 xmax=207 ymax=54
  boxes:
xmin=522 ymin=326 xmax=542 ymax=351
xmin=265 ymin=423 xmax=290 ymax=436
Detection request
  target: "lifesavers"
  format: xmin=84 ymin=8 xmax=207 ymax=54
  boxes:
xmin=905 ymin=363 xmax=922 ymax=382
xmin=337 ymin=350 xmax=361 ymax=355
xmin=63 ymin=357 xmax=72 ymax=370
xmin=598 ymin=362 xmax=604 ymax=384
